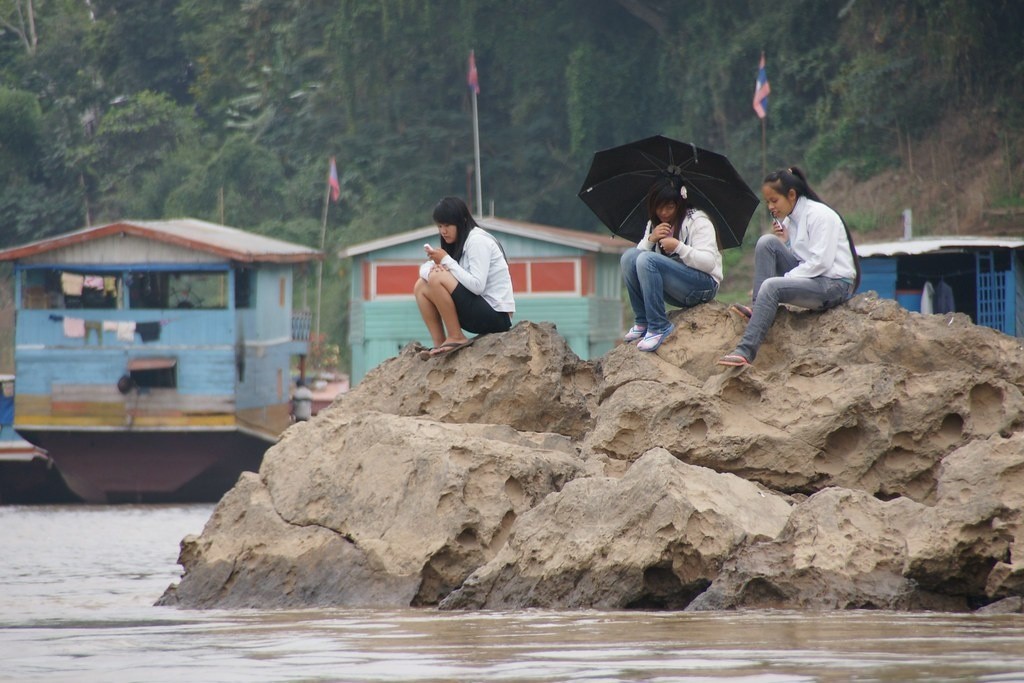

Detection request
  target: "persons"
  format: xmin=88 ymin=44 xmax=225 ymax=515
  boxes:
xmin=620 ymin=178 xmax=723 ymax=352
xmin=718 ymin=164 xmax=860 ymax=367
xmin=413 ymin=195 xmax=516 ymax=356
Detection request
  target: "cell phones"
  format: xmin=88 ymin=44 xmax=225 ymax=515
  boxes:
xmin=770 ymin=211 xmax=783 ymax=230
xmin=424 ymin=244 xmax=433 ymax=254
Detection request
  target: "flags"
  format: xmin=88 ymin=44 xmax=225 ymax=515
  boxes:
xmin=752 ymin=55 xmax=772 ymax=119
xmin=327 ymin=155 xmax=340 ymax=203
xmin=467 ymin=48 xmax=480 ymax=95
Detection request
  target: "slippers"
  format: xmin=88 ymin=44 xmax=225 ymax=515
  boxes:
xmin=419 ymin=338 xmax=473 ymax=361
xmin=623 ymin=325 xmax=647 ymax=341
xmin=717 ymin=354 xmax=750 ymax=365
xmin=637 ymin=322 xmax=674 ymax=353
xmin=730 ymin=304 xmax=753 ymax=321
